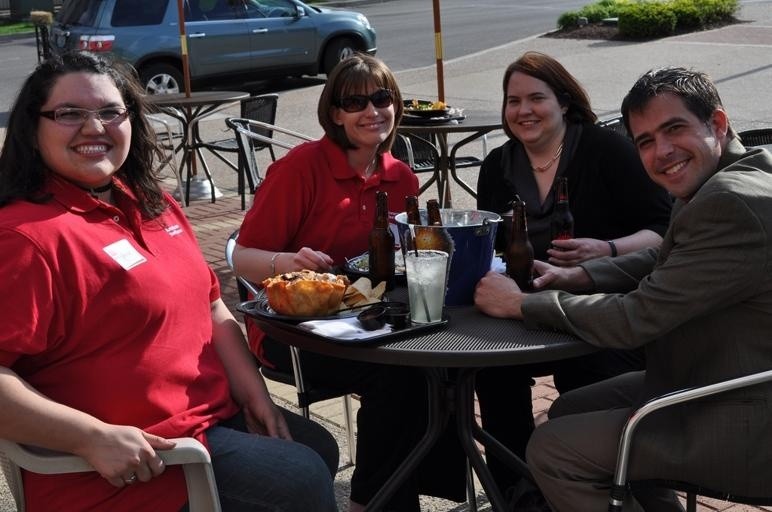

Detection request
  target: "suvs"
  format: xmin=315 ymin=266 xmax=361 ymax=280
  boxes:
xmin=44 ymin=0 xmax=379 ymax=108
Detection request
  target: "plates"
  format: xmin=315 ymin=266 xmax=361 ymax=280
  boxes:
xmin=343 ymin=256 xmax=405 ymax=277
xmin=409 ymin=109 xmax=451 ymax=118
xmin=256 ymin=288 xmax=361 ymax=319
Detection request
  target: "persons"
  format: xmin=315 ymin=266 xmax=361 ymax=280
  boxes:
xmin=478 ymin=52 xmax=671 ymax=511
xmin=0 ymin=50 xmax=341 ymax=510
xmin=474 ymin=67 xmax=771 ymax=511
xmin=232 ymin=54 xmax=467 ymax=512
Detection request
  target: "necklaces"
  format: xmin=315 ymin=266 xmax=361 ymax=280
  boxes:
xmin=524 ymin=143 xmax=567 ymax=173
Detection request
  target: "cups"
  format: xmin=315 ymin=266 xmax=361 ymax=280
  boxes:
xmin=357 ymin=301 xmax=411 ymax=330
xmin=404 ymin=250 xmax=449 ymax=323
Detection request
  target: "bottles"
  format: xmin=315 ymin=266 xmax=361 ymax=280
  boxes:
xmin=403 ymin=194 xmax=421 ymax=225
xmin=426 ymin=199 xmax=443 ymax=226
xmin=505 ymin=202 xmax=535 ymax=292
xmin=547 ymin=177 xmax=574 ymax=265
xmin=368 ymin=192 xmax=395 ymax=292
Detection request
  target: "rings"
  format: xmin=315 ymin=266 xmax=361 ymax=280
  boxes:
xmin=148 ymin=458 xmax=164 ymax=472
xmin=123 ymin=471 xmax=139 ymax=485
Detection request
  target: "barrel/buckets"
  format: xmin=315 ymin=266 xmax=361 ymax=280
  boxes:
xmin=394 ymin=208 xmax=505 ymax=307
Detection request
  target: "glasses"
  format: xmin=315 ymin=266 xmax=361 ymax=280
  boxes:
xmin=38 ymin=105 xmax=130 ymax=125
xmin=335 ymin=89 xmax=394 ymax=113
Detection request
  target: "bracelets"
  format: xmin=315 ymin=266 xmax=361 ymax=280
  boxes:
xmin=600 ymin=233 xmax=623 ymax=264
xmin=267 ymin=249 xmax=286 ymax=282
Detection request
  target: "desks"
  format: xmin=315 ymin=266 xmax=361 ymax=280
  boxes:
xmin=394 ymin=110 xmax=507 ymax=211
xmin=115 ymin=91 xmax=286 ymax=208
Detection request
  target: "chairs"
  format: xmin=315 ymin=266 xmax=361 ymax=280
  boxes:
xmin=191 ymin=94 xmax=280 ymax=187
xmin=0 ymin=424 xmax=231 ymax=512
xmin=594 ymin=366 xmax=772 ymax=509
xmin=391 ymin=98 xmax=490 ymax=172
xmin=222 ymin=209 xmax=369 ymax=472
